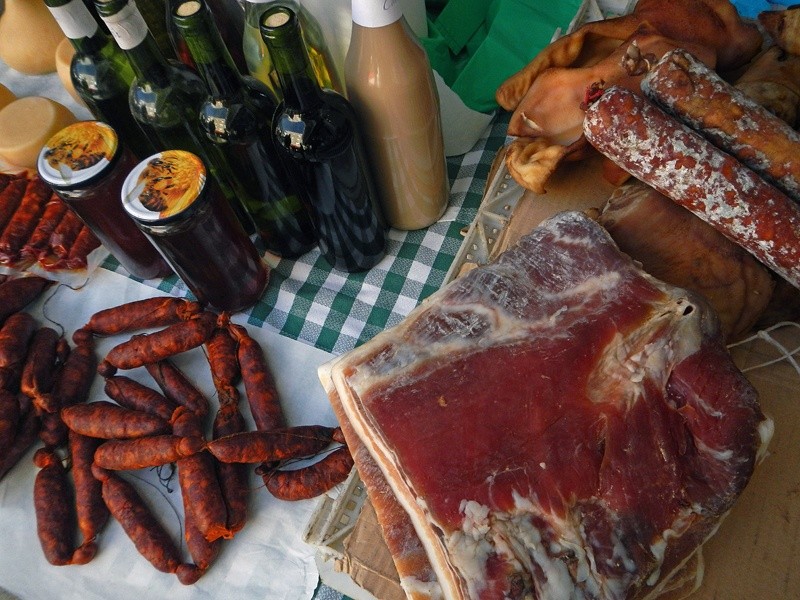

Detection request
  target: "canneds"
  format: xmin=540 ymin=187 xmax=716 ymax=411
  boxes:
xmin=38 ymin=122 xmax=175 ymax=280
xmin=122 ymin=151 xmax=270 ymax=316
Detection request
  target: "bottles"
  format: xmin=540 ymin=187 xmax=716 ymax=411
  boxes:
xmin=42 ymin=0 xmax=449 ymax=271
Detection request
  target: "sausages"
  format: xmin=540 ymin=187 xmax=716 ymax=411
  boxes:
xmin=578 ymin=39 xmax=800 ymax=289
xmin=0 ymin=277 xmax=355 ymax=585
xmin=1 ymin=169 xmax=101 ymax=269
